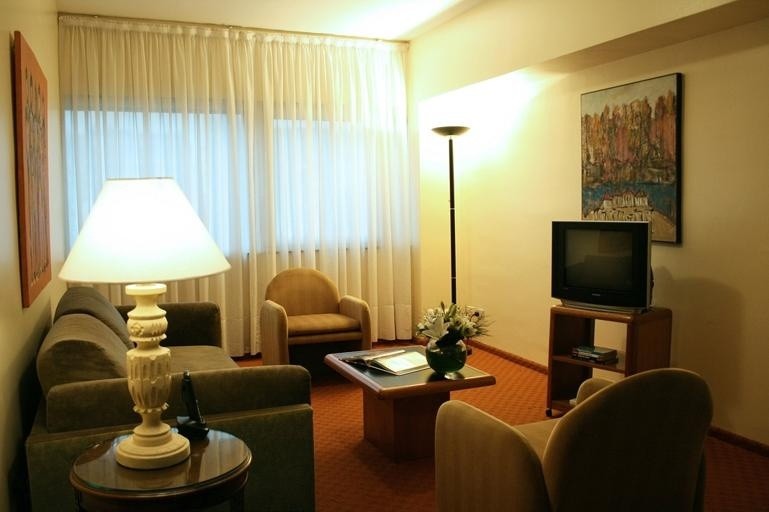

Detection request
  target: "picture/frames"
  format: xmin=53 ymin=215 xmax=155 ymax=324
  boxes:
xmin=14 ymin=28 xmax=54 ymax=309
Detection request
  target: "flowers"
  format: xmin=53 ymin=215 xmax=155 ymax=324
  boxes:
xmin=415 ymin=300 xmax=491 ymax=344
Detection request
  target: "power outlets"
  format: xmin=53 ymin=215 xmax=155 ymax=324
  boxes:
xmin=465 ymin=305 xmax=484 ymax=321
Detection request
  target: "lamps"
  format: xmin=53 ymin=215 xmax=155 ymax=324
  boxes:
xmin=56 ymin=177 xmax=232 ymax=470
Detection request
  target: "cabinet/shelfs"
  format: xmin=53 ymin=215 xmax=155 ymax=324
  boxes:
xmin=545 ymin=305 xmax=673 ymax=418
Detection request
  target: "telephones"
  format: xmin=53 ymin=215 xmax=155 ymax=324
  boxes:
xmin=176 ymin=370 xmax=210 ymax=438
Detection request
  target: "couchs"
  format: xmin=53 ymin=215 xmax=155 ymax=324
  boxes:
xmin=25 ymin=286 xmax=314 ymax=511
xmin=260 ymin=268 xmax=372 ymax=364
xmin=434 ymin=368 xmax=714 ymax=512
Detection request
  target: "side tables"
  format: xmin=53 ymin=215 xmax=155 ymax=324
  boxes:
xmin=69 ymin=426 xmax=253 ymax=510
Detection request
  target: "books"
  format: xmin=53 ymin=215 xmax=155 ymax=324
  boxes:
xmin=568 ymin=398 xmax=577 ymax=406
xmin=339 ymin=348 xmax=430 ymax=376
xmin=572 ymin=346 xmax=619 ymax=365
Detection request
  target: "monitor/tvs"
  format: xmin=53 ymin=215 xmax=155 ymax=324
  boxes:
xmin=551 ymin=220 xmax=657 ymax=315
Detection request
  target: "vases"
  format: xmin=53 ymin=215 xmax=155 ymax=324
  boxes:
xmin=426 ymin=339 xmax=467 ymax=374
